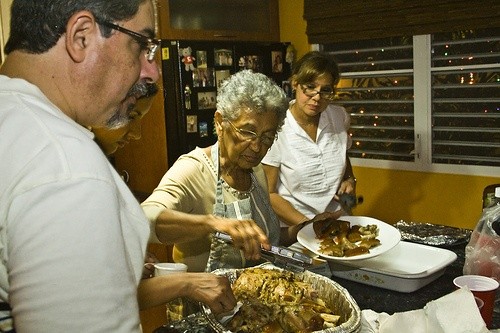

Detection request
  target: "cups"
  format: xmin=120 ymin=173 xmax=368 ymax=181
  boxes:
xmin=151 ymin=262 xmax=188 ymax=280
xmin=472 ymin=221 xmax=500 ymax=281
xmin=453 ymin=275 xmax=499 ymax=330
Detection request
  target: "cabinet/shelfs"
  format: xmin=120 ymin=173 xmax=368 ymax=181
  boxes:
xmin=155 ymin=0 xmax=281 ymax=41
xmin=162 ymin=38 xmax=295 ymax=170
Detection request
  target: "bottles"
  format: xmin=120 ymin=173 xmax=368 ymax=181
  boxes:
xmin=482 ymin=183 xmax=500 ymax=234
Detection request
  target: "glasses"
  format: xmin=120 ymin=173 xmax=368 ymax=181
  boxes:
xmin=93 ymin=17 xmax=161 ymax=62
xmin=227 ymin=118 xmax=279 ymax=145
xmin=300 ymin=83 xmax=335 ymax=100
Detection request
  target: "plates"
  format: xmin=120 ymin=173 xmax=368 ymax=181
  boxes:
xmin=391 ymin=220 xmax=473 ymax=248
xmin=297 ymin=215 xmax=401 ymax=261
xmin=286 ymin=240 xmax=457 ymax=293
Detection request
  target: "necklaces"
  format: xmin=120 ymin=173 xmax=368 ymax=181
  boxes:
xmin=222 ymin=162 xmax=250 ymax=198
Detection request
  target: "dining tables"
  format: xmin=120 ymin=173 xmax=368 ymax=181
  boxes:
xmin=153 ymin=244 xmax=500 ymax=333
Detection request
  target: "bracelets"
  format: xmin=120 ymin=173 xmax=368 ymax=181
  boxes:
xmin=300 ymin=221 xmax=308 ymax=232
xmin=345 ymin=175 xmax=357 ymax=182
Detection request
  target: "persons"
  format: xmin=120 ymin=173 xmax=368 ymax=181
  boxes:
xmin=259 ymin=50 xmax=357 ymax=241
xmin=0 ymin=0 xmax=162 ymax=333
xmin=83 ymin=76 xmax=237 ymax=314
xmin=138 ymin=70 xmax=343 ymax=277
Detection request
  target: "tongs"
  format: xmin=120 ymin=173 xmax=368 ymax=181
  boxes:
xmin=215 ymin=232 xmax=313 ymax=273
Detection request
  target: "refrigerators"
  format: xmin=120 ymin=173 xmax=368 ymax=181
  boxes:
xmin=161 ymin=35 xmax=295 ymax=167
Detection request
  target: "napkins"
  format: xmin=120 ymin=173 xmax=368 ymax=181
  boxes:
xmin=362 ymin=285 xmax=489 ymax=333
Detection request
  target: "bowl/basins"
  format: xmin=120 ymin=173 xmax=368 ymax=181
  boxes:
xmin=202 ymin=265 xmax=361 ymax=333
